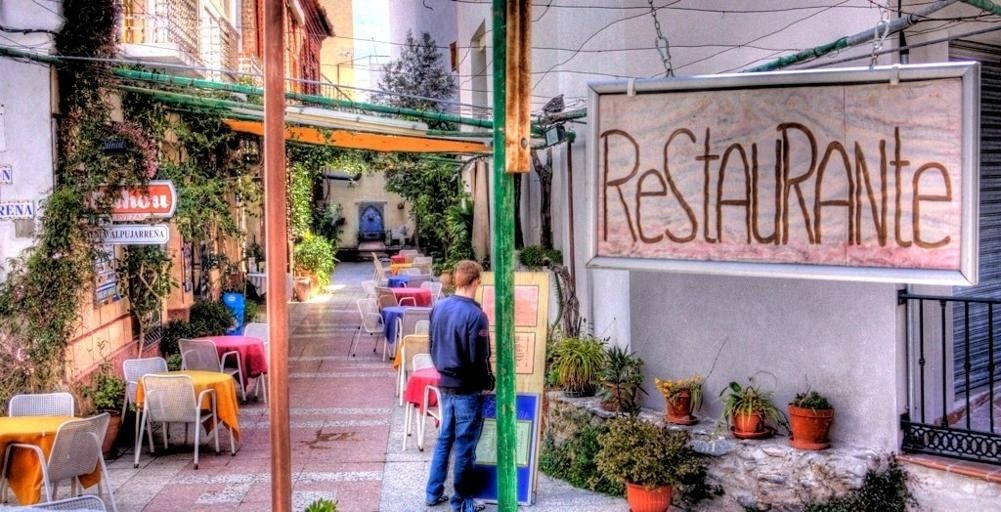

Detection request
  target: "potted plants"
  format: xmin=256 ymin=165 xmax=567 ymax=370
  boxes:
xmin=653 ymin=365 xmax=705 ymax=426
xmin=598 ymin=411 xmax=703 ymax=512
xmin=600 ymin=343 xmax=649 ymax=414
xmin=783 ymin=381 xmax=835 ymax=451
xmin=717 ymin=376 xmax=791 ymax=442
xmin=544 ymin=329 xmax=611 ymax=403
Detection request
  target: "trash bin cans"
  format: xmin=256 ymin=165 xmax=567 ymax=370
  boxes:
xmin=222 ymin=292 xmax=245 ymax=335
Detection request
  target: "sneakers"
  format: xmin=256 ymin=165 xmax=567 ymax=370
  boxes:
xmin=426 ymin=494 xmax=448 ymax=507
xmin=456 ymin=504 xmax=485 ymax=512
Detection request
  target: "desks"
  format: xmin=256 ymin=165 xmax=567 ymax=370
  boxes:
xmin=133 ymin=370 xmax=241 ymax=457
xmin=189 ymin=335 xmax=268 ymax=395
xmin=0 ymin=415 xmax=102 ymax=507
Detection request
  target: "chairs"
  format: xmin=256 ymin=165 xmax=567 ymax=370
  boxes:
xmin=238 ymin=256 xmax=267 ymax=301
xmin=119 ymin=357 xmax=177 ymax=449
xmin=2 ymin=411 xmax=117 ymax=512
xmin=8 ymin=392 xmax=75 ymax=503
xmin=346 ymin=248 xmax=451 ymax=451
xmin=21 ymin=493 xmax=106 ymax=510
xmin=132 ymin=373 xmax=220 ymax=470
xmin=253 ymin=342 xmax=269 ymax=404
xmin=177 ymin=338 xmax=247 ymax=404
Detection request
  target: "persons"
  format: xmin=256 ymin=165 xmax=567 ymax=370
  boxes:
xmin=424 ymin=258 xmax=497 ymax=512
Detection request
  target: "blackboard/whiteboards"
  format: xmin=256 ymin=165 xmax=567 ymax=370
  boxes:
xmin=469 ymin=392 xmax=542 ymax=505
